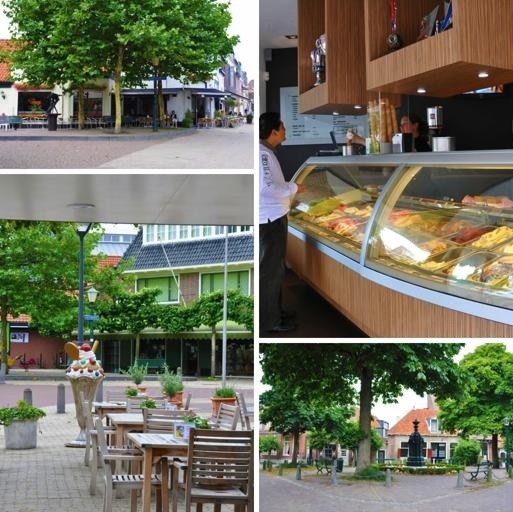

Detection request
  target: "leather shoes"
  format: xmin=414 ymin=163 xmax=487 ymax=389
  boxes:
xmin=261 ymin=320 xmax=300 ymax=332
xmin=267 ymin=308 xmax=297 ymax=319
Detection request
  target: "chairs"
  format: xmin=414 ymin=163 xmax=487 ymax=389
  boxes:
xmin=1 ymin=113 xmax=245 ymax=131
xmin=76 ymin=389 xmax=252 ymax=512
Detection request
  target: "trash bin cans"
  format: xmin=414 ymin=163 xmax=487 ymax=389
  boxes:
xmin=336 ymin=458 xmax=344 ymax=472
xmin=46 ymin=113 xmax=61 ymax=131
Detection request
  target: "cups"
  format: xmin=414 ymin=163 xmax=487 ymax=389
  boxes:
xmin=402 ymin=134 xmax=414 ymax=153
xmin=365 ymin=137 xmax=372 ymax=154
xmin=379 ymin=142 xmax=401 ymax=154
xmin=342 ymin=144 xmax=363 ymax=155
xmin=431 ymin=137 xmax=458 ymax=152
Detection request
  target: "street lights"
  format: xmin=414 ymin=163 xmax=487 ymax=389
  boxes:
xmin=72 ymin=219 xmax=93 ymax=349
xmin=85 ymin=284 xmax=98 ymax=354
xmin=501 ymin=416 xmax=510 ymax=480
xmin=148 ymin=53 xmax=161 ymax=133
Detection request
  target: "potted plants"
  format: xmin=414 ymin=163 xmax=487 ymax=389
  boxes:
xmin=0 ymin=400 xmax=46 ymax=452
xmin=156 ymin=364 xmax=184 ymax=405
xmin=211 ymin=379 xmax=238 ymax=416
xmin=120 ymin=359 xmax=148 ymax=392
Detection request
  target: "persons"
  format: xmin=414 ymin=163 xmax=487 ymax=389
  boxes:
xmin=347 ymin=114 xmax=429 ymax=153
xmin=169 ymin=110 xmax=175 ymax=127
xmin=259 ymin=110 xmax=306 ymax=333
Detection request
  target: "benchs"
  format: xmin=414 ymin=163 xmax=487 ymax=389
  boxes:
xmin=129 ymin=359 xmax=166 ymax=373
xmin=467 ymin=461 xmax=495 ymax=480
xmin=313 ymin=458 xmax=334 ymax=475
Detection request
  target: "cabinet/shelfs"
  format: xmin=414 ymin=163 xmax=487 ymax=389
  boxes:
xmin=362 ymin=1 xmax=512 ymax=100
xmin=288 ymin=150 xmax=513 ymax=338
xmin=295 ymin=0 xmax=401 ymax=115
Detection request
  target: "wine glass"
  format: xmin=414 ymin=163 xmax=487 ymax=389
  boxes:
xmin=307 ymin=34 xmax=325 ymax=86
